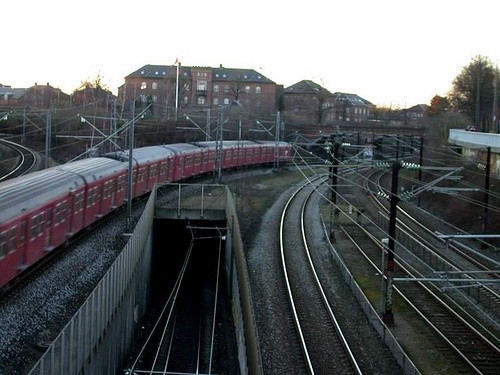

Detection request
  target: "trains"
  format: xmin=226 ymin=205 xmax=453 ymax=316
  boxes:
xmin=0 ymin=139 xmax=290 ymax=293
